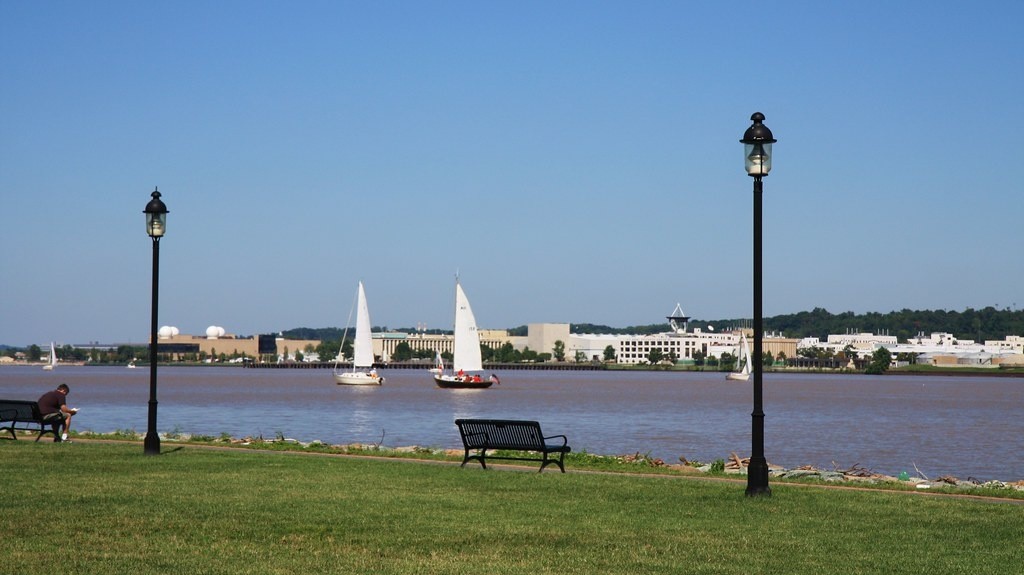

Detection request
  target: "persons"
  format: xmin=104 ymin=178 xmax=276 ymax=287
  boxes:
xmin=463 ymin=375 xmax=470 ymax=383
xmin=370 ymin=367 xmax=377 ymax=380
xmin=37 ymin=384 xmax=78 ymax=444
xmin=473 ymin=375 xmax=481 ymax=383
xmin=438 ymin=364 xmax=443 ymax=379
xmin=457 ymin=369 xmax=465 ymax=380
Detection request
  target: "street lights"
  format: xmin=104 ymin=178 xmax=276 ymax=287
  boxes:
xmin=143 ymin=187 xmax=171 ymax=456
xmin=737 ymin=109 xmax=779 ymax=498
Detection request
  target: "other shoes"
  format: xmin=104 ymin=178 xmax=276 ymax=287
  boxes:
xmin=62 ymin=437 xmax=73 ymax=444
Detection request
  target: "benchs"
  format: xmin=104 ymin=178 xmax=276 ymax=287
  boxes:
xmin=455 ymin=418 xmax=571 ymax=473
xmin=0 ymin=399 xmax=65 ymax=443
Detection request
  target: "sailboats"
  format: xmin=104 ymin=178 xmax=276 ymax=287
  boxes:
xmin=433 ymin=266 xmax=499 ymax=388
xmin=334 ymin=279 xmax=383 ymax=385
xmin=726 ymin=329 xmax=752 ymax=382
xmin=428 ymin=349 xmax=444 ymax=373
xmin=41 ymin=342 xmax=59 ymax=371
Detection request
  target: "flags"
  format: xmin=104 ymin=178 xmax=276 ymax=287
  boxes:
xmin=489 ymin=373 xmax=501 ymax=385
